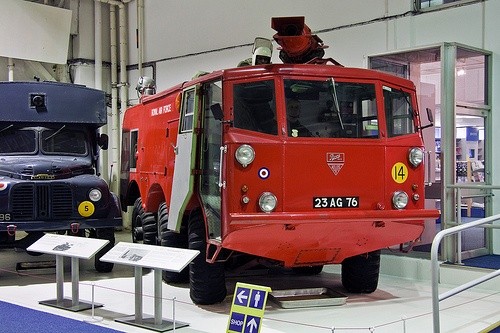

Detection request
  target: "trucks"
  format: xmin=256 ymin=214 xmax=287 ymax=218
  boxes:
xmin=1 ymin=75 xmax=117 ymax=274
xmin=119 ymin=11 xmax=430 ymax=305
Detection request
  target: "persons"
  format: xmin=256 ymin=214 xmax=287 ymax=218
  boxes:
xmin=286 ymin=99 xmax=314 ymax=137
xmin=317 ymin=100 xmax=340 ymax=122
xmin=237 ymin=46 xmax=278 ymax=134
